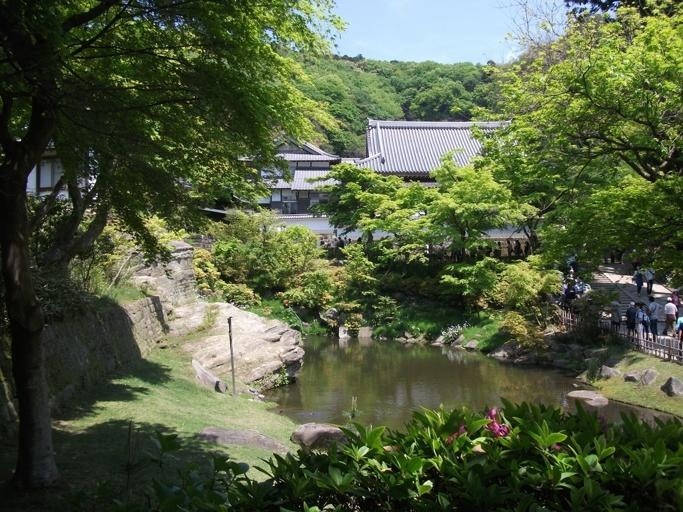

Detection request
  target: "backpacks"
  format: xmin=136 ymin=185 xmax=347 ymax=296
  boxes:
xmin=637 ymin=309 xmax=651 ymax=328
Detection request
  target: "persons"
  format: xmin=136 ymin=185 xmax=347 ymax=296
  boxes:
xmin=424 ymin=237 xmax=532 ymax=264
xmin=316 ymin=234 xmax=361 ymax=252
xmin=644 ymin=266 xmax=656 ymax=296
xmin=630 ymin=265 xmax=644 ymax=297
xmin=554 ymin=275 xmax=682 ymax=362
